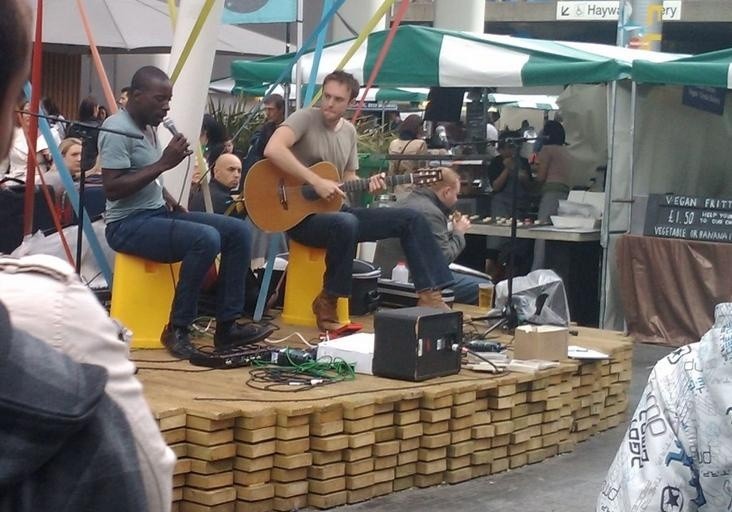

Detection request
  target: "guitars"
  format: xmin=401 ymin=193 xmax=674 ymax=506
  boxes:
xmin=243 ymin=158 xmax=443 ymax=234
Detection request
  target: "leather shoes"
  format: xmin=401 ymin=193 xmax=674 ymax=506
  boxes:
xmin=160 ymin=323 xmax=199 ymax=359
xmin=311 ymin=290 xmax=342 ymax=333
xmin=416 ymin=290 xmax=472 ymax=323
xmin=213 ymin=322 xmax=272 ymax=350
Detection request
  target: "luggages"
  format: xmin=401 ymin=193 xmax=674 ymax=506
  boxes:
xmin=0 ymin=176 xmax=57 ymax=255
xmin=60 ymin=174 xmax=106 ymax=225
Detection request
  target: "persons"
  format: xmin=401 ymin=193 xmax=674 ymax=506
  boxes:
xmin=381 ymin=167 xmax=492 ymax=306
xmin=187 ymin=94 xmax=288 ymax=260
xmin=0 ymin=0 xmax=179 ymax=512
xmin=384 ymin=105 xmax=571 ymax=226
xmin=263 ymin=70 xmax=455 ymax=333
xmin=0 ymin=86 xmax=131 ymax=194
xmin=595 ymin=302 xmax=732 ymax=512
xmin=97 ymin=66 xmax=276 ymax=359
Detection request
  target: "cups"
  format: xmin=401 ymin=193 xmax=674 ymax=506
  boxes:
xmin=478 ymin=281 xmax=494 ymax=311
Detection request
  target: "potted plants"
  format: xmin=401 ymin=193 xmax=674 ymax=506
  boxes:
xmin=330 ymin=115 xmax=392 ymax=204
xmin=198 ymin=84 xmax=273 ymax=182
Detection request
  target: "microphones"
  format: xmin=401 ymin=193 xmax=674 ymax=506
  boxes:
xmin=451 ymin=344 xmax=470 ymax=354
xmin=161 ymin=114 xmax=188 ymax=152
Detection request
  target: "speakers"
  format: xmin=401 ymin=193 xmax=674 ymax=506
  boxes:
xmin=373 ymin=305 xmax=464 ymax=380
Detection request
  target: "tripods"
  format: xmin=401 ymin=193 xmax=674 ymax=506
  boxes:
xmin=471 ymin=143 xmax=578 ymax=341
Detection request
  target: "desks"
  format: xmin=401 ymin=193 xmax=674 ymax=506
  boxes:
xmin=447 ymin=210 xmax=602 ymax=289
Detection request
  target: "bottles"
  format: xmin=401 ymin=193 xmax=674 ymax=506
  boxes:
xmin=390 ymin=258 xmax=409 ymax=284
